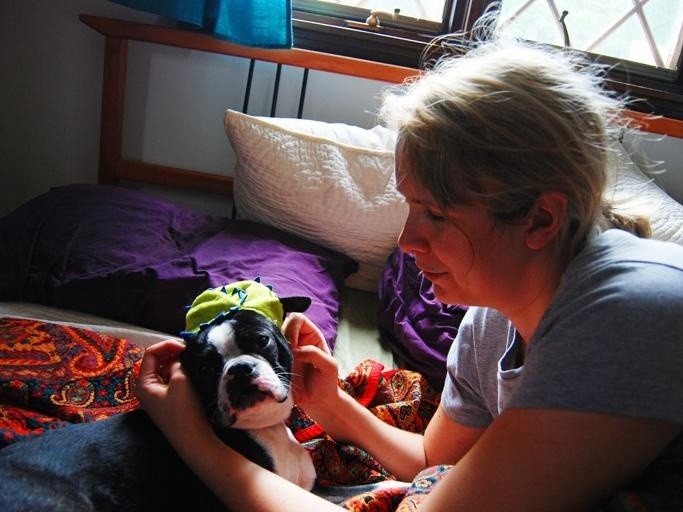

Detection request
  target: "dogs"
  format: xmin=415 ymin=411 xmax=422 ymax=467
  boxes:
xmin=0 ymin=295 xmax=317 ymax=512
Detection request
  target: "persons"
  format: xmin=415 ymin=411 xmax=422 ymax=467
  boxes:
xmin=132 ymin=3 xmax=683 ymax=512
